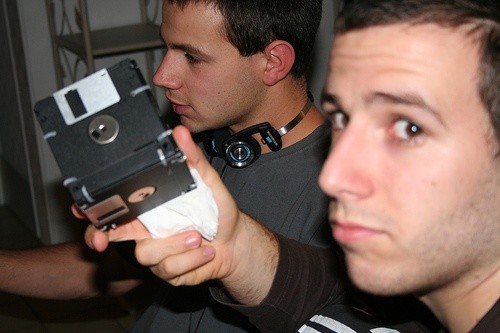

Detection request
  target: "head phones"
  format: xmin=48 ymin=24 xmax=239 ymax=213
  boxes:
xmin=208 ymin=90 xmax=313 ymax=168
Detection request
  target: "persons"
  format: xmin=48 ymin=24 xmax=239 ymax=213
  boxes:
xmin=0 ymin=0 xmax=391 ymax=333
xmin=71 ymin=1 xmax=500 ymax=333
